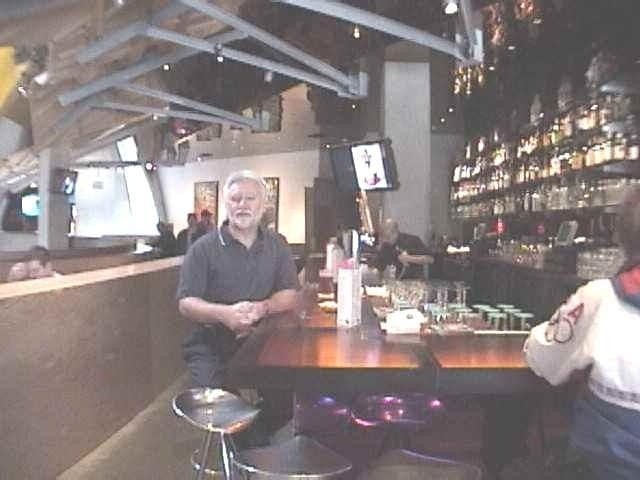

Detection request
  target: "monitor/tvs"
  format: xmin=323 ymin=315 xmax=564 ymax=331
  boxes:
xmin=51 ymin=168 xmax=78 ymax=195
xmin=348 ymin=138 xmax=399 ymax=192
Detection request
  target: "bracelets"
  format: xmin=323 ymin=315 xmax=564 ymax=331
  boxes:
xmin=261 ymin=302 xmax=273 ymax=320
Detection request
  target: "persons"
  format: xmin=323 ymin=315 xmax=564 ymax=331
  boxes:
xmin=375 ymin=218 xmax=435 ymax=278
xmin=172 ymin=169 xmax=304 ymax=450
xmin=199 ymin=209 xmax=214 ymax=230
xmin=151 ymin=221 xmax=177 ymax=259
xmin=177 ymin=213 xmax=197 ymax=254
xmin=359 ymin=149 xmax=381 ymax=186
xmin=520 ymin=182 xmax=640 ymax=479
xmin=6 ymin=243 xmax=52 ymax=284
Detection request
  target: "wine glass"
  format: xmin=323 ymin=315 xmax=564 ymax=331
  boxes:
xmin=393 ymin=279 xmax=475 ymax=335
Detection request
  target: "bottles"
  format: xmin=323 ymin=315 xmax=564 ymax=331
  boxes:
xmin=326 ymin=237 xmax=342 ymax=273
xmin=451 ymin=93 xmax=640 ymax=218
xmin=472 ymin=223 xmax=553 ymax=269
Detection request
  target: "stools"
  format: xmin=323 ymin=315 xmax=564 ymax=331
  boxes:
xmin=170 ymin=385 xmax=359 ymax=480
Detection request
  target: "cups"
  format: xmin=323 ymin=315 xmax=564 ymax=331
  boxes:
xmin=424 ymin=303 xmax=536 ymax=331
xmin=575 ymin=249 xmax=625 ymax=280
